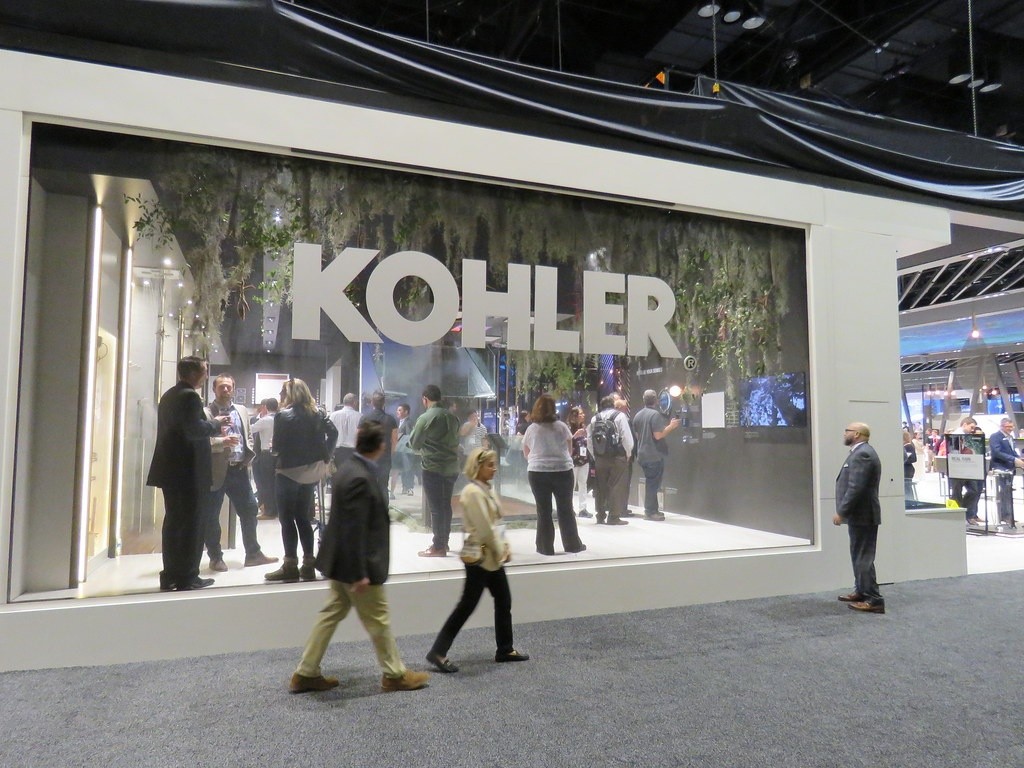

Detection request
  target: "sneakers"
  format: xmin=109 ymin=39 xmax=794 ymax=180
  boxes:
xmin=289 ymin=671 xmax=340 ymax=693
xmin=381 ymin=670 xmax=431 ymax=691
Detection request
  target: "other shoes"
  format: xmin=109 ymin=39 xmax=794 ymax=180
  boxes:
xmin=160 ymin=579 xmax=177 ymax=591
xmin=967 ymin=514 xmax=1017 ymax=525
xmin=609 ymin=519 xmax=629 ymax=524
xmin=597 ymin=520 xmax=605 ymax=524
xmin=209 ymin=558 xmax=228 ymax=572
xmin=618 ymin=510 xmax=635 ymax=517
xmin=645 ymin=512 xmax=665 ymax=521
xmin=494 ymin=649 xmax=530 ymax=663
xmin=579 ymin=509 xmax=593 ymax=518
xmin=417 ymin=544 xmax=449 ymax=557
xmin=178 ymin=576 xmax=216 ymax=591
xmin=425 ymin=650 xmax=459 ymax=672
xmin=243 ymin=552 xmax=279 ymax=567
xmin=257 ymin=482 xmax=422 ymax=520
xmin=571 ymin=544 xmax=586 ymax=552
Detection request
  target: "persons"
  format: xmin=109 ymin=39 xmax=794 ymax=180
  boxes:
xmin=143 ymin=357 xmax=684 ymax=591
xmin=903 ymin=416 xmax=1024 ymax=530
xmin=288 ymin=420 xmax=430 ymax=696
xmin=426 ymin=446 xmax=528 ymax=674
xmin=832 ymin=422 xmax=886 ymax=615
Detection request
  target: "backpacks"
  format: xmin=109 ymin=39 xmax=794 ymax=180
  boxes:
xmin=593 ymin=411 xmax=621 ymax=457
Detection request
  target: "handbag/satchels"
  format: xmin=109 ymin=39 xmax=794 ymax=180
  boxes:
xmin=571 ymin=436 xmax=593 ymax=467
xmin=459 ymin=540 xmax=485 ymax=564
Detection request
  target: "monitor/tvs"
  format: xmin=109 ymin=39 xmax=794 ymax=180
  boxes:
xmin=738 ymin=372 xmax=807 ymax=427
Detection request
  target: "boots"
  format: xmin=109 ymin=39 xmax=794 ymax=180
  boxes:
xmin=298 ymin=556 xmax=316 ymax=579
xmin=264 ymin=557 xmax=300 ymax=582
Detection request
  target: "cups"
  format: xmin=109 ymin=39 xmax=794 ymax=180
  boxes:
xmin=228 ymin=435 xmax=242 ymax=453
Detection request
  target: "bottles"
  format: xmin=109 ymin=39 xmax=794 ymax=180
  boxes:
xmin=612 ymin=435 xmax=617 ymax=445
xmin=590 ymin=461 xmax=595 ymax=478
xmin=219 ymin=408 xmax=231 ymax=435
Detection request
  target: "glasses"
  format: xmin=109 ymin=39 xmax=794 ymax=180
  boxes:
xmin=845 ymin=428 xmax=858 ymax=433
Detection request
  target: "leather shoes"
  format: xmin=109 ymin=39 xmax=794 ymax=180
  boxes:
xmin=838 ymin=590 xmax=867 ymax=602
xmin=849 ymin=601 xmax=882 ymax=612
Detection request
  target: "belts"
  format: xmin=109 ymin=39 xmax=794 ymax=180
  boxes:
xmin=228 ymin=461 xmax=242 ymax=467
